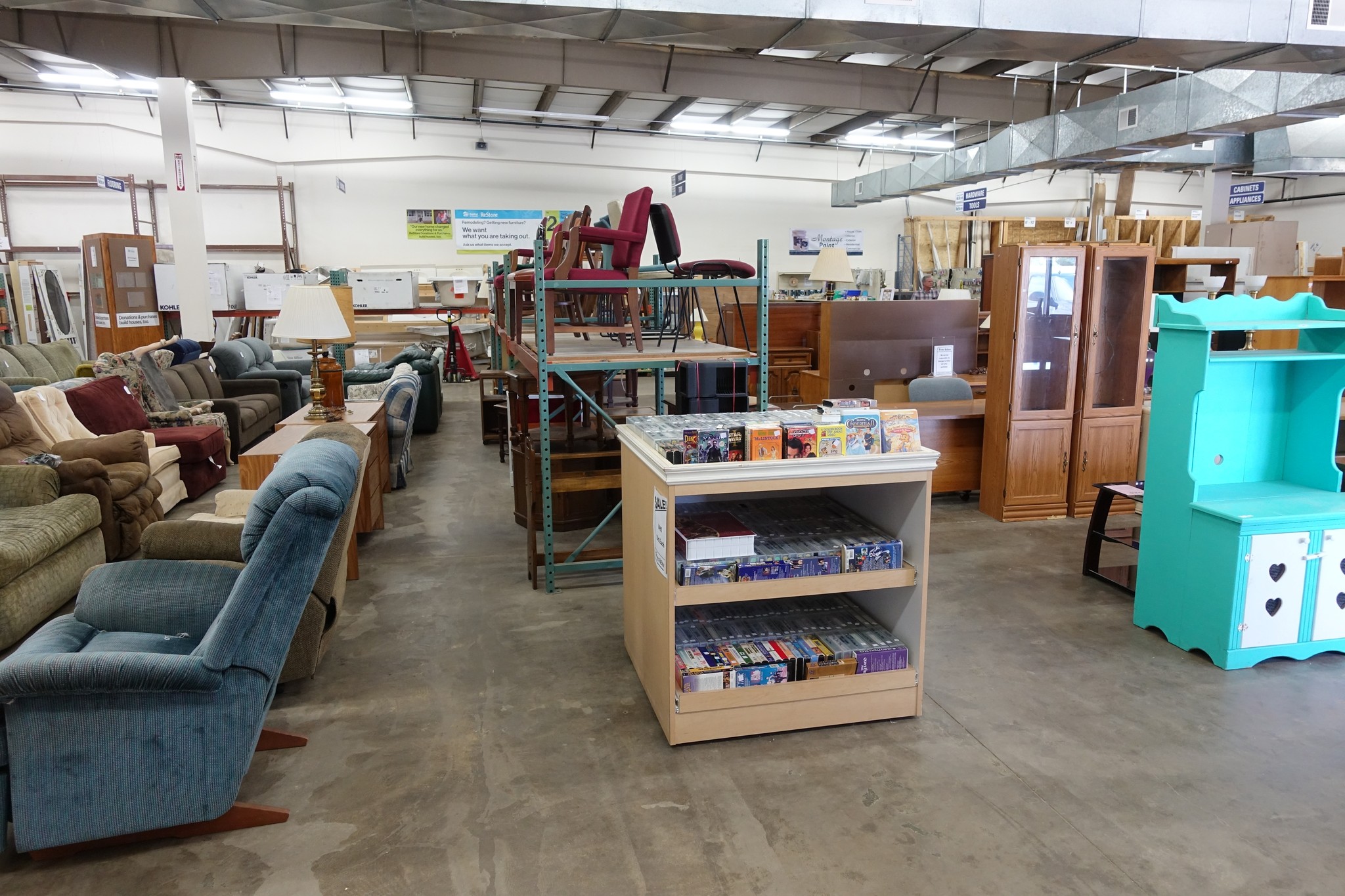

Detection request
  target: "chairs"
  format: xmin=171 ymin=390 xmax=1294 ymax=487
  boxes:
xmin=937 ymin=287 xmax=971 ymax=300
xmin=485 ymin=185 xmax=757 ymax=354
xmin=906 ymin=377 xmax=975 ymax=400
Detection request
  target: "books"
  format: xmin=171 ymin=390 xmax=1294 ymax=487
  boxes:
xmin=745 ymin=425 xmax=783 ymax=461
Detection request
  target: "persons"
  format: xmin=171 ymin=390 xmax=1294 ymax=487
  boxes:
xmin=717 ymin=568 xmax=732 ymax=579
xmin=802 ymin=443 xmax=816 ymax=458
xmin=695 ymin=565 xmax=715 ymax=578
xmin=699 ymin=440 xmax=728 ymax=463
xmin=787 ymin=438 xmax=804 ymax=458
xmin=911 ymin=274 xmax=940 ymax=300
xmin=729 ymin=452 xmax=743 ymax=461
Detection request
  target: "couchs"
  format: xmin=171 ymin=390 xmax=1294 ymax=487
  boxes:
xmin=0 ymin=335 xmax=448 ymax=875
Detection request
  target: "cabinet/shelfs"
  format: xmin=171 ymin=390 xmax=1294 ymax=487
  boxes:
xmin=275 ymin=399 xmax=390 ymax=498
xmin=614 ymin=423 xmax=940 ymax=747
xmin=977 ymin=212 xmax=1345 ymax=671
xmin=237 ymin=422 xmax=389 ymax=582
xmin=480 ymin=238 xmax=772 ymax=588
xmin=737 ymin=344 xmax=815 ymax=402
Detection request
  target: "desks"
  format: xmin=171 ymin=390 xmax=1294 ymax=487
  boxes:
xmin=873 ymin=398 xmax=986 ymax=500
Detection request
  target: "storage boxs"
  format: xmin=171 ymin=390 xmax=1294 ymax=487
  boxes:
xmin=344 ymin=341 xmax=416 ymax=370
xmin=346 ymin=270 xmax=421 ymax=311
xmin=243 ymin=272 xmax=318 ymax=311
xmin=152 ymin=261 xmax=246 ymax=312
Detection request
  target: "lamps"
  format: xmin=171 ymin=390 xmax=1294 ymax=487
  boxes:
xmin=1236 ymin=273 xmax=1267 ymax=350
xmin=806 ymin=247 xmax=855 ymax=301
xmin=272 ymin=285 xmax=359 ymax=420
xmin=1199 ymin=274 xmax=1227 ymax=352
